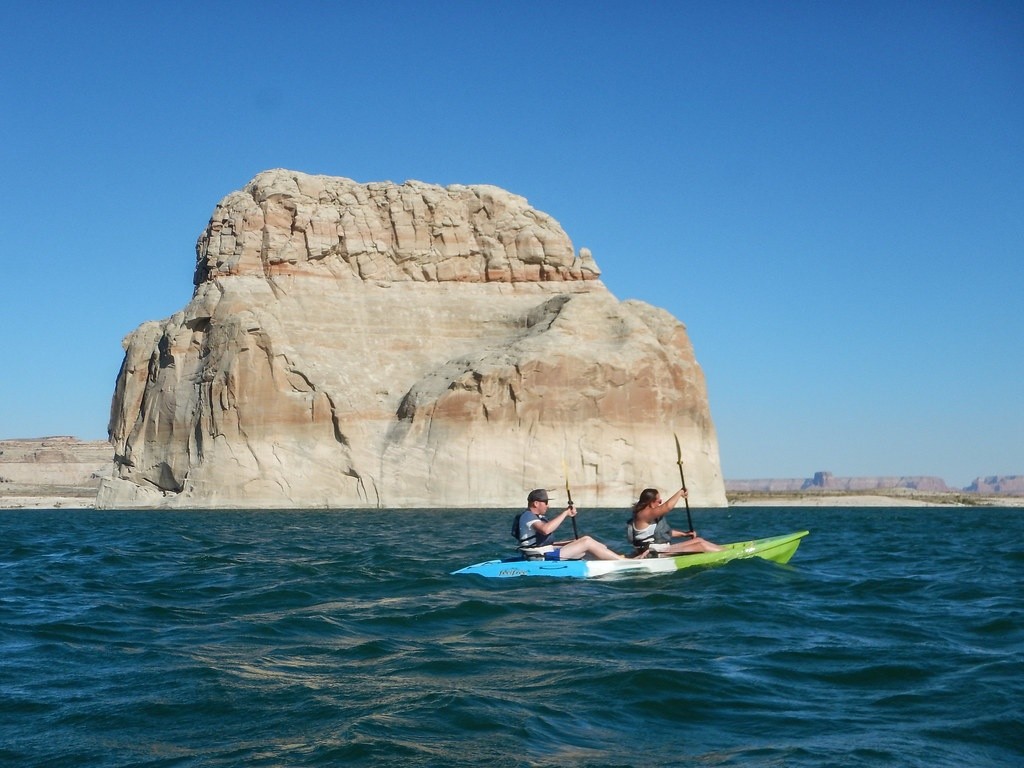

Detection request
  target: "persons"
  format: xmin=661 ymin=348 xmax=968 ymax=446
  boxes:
xmin=626 ymin=487 xmax=728 ymax=556
xmin=511 ymin=489 xmax=649 ymax=560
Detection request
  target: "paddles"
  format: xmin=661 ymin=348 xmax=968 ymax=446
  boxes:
xmin=674 ymin=431 xmax=696 ymax=541
xmin=564 ymin=471 xmax=578 ymax=538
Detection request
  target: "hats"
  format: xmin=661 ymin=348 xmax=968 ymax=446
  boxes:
xmin=528 ymin=489 xmax=554 ymax=502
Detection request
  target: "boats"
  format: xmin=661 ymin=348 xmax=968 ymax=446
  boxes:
xmin=449 ymin=531 xmax=810 ymax=586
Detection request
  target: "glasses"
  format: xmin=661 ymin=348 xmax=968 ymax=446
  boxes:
xmin=654 ymin=499 xmax=661 ymax=504
